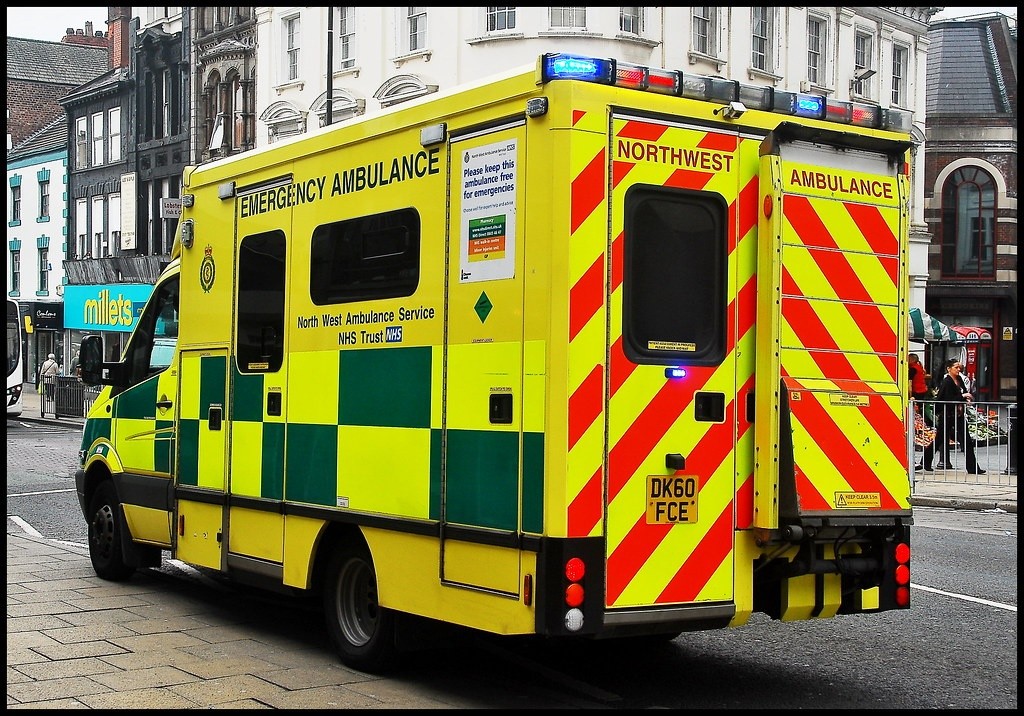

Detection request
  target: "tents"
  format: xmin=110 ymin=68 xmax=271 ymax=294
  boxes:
xmin=907 ymin=307 xmax=968 ymax=397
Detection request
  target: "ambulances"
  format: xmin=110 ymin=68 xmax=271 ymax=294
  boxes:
xmin=74 ymin=55 xmax=919 ymax=680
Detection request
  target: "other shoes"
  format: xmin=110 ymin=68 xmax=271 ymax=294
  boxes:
xmin=919 ymin=461 xmax=933 ymax=472
xmin=967 ymin=469 xmax=985 ymax=474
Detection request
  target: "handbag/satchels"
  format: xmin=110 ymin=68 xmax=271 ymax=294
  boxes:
xmin=38 ymin=381 xmax=46 ymax=395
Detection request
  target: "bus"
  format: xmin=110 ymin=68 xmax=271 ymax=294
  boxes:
xmin=7 ymin=299 xmax=37 ymax=416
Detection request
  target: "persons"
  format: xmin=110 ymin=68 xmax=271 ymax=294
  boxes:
xmin=40 ymin=353 xmax=58 ymax=403
xmin=70 ymin=349 xmax=80 ymax=376
xmin=920 ymin=359 xmax=986 ymax=475
xmin=908 ymin=353 xmax=938 ymax=430
